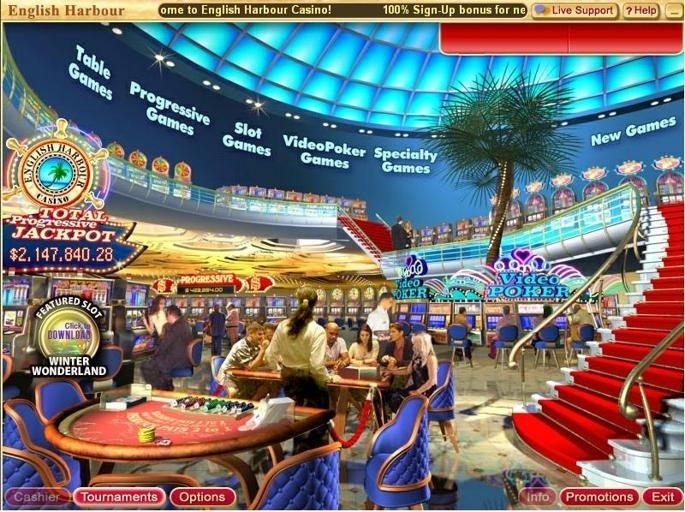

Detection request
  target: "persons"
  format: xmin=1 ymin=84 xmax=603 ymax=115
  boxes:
xmin=390 ymin=216 xmax=414 ymax=250
xmin=566 ymin=304 xmax=596 ymax=350
xmin=531 ymin=304 xmax=562 ymax=359
xmin=488 ymin=304 xmax=521 ymax=359
xmin=142 ymin=285 xmax=438 ymax=456
xmin=447 ymin=307 xmax=472 ymax=361
